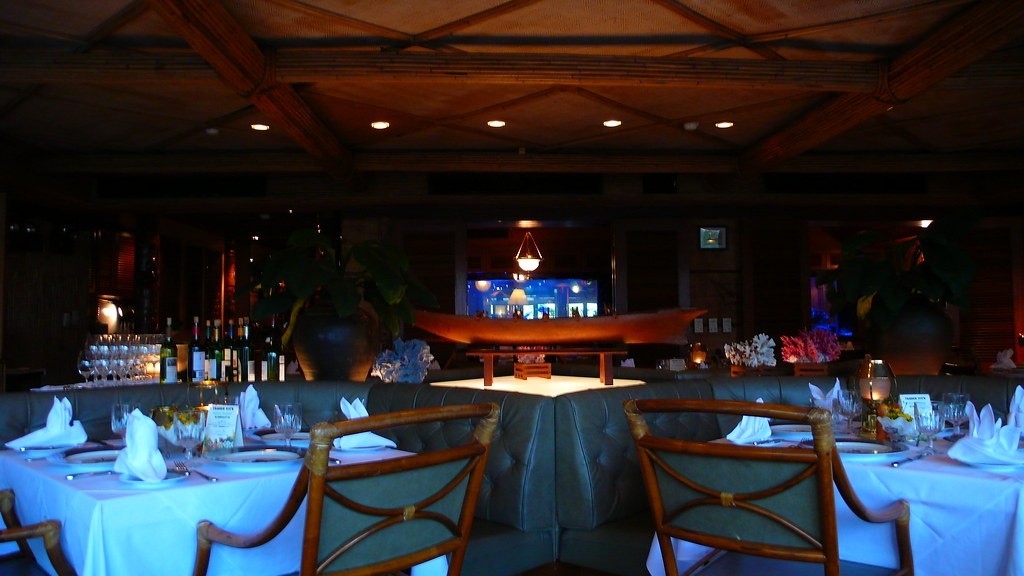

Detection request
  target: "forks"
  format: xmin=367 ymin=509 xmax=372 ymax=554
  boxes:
xmin=174 ymin=459 xmax=218 ymax=482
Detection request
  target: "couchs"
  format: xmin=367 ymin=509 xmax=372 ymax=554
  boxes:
xmin=0 ymin=378 xmax=557 ymax=576
xmin=557 ymin=375 xmax=1024 ymax=576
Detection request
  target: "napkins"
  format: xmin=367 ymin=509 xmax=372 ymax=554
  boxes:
xmin=1009 ymin=384 xmax=1024 ymax=414
xmin=114 ymin=408 xmax=166 ymax=482
xmin=4 ymin=396 xmax=88 ymax=450
xmin=947 ymin=384 xmax=1024 ymax=465
xmin=726 ymin=396 xmax=772 ymax=445
xmin=807 ymin=378 xmax=847 ymax=414
xmin=332 ymin=397 xmax=396 ymax=449
xmin=233 ymin=385 xmax=272 ymax=430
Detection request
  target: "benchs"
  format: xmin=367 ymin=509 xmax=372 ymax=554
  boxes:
xmin=465 ymin=348 xmax=629 ymax=385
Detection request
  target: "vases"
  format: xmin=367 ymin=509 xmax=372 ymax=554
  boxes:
xmin=395 ymin=369 xmax=427 ymax=385
xmin=794 ymin=361 xmax=829 ymax=377
xmin=731 ymin=365 xmax=770 ymax=378
xmin=514 ymin=362 xmax=551 ymax=380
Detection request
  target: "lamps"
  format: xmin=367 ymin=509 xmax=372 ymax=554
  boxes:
xmin=514 ymin=228 xmax=545 ymax=276
xmin=507 ymin=288 xmax=529 ymax=319
xmin=689 ymin=343 xmax=708 ymax=369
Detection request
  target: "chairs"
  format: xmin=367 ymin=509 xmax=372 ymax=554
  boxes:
xmin=193 ymin=401 xmax=503 ymax=576
xmin=0 ymin=488 xmax=78 ymax=576
xmin=624 ymin=398 xmax=916 ymax=576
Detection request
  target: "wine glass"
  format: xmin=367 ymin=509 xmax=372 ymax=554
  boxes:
xmin=173 ymin=410 xmax=206 ymax=468
xmin=837 ymin=390 xmax=863 ymax=436
xmin=77 ymin=334 xmax=164 ymax=388
xmin=915 ymin=392 xmax=970 ymax=454
xmin=111 ymin=401 xmax=140 ymax=446
xmin=274 ymin=402 xmax=302 ymax=446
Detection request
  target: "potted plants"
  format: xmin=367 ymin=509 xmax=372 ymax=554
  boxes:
xmin=810 ymin=217 xmax=977 ymax=376
xmin=235 ymin=228 xmax=441 ymax=384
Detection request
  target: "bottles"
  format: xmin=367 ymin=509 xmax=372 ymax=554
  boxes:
xmin=160 ymin=318 xmax=177 ymax=384
xmin=187 ymin=317 xmax=285 ymax=382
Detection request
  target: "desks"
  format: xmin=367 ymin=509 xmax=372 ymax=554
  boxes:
xmin=646 ymin=421 xmax=1024 ymax=576
xmin=0 ymin=383 xmax=450 ymax=576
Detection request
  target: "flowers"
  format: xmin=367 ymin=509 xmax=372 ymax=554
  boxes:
xmin=370 ymin=337 xmax=440 ymax=383
xmin=780 ymin=326 xmax=841 ymax=363
xmin=723 ymin=333 xmax=776 ymax=368
xmin=516 ymin=346 xmax=548 ymax=364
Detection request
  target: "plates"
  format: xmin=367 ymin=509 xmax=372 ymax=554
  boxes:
xmin=216 ymin=451 xmax=301 ymax=468
xmin=799 ymin=438 xmax=910 ymax=454
xmin=119 ymin=468 xmax=189 ymax=487
xmin=252 ymin=428 xmax=311 ymax=447
xmin=769 ymin=425 xmax=814 ymax=437
xmin=13 ymin=441 xmax=74 ymax=458
xmin=332 ymin=446 xmax=385 ymax=451
xmin=47 ymin=445 xmax=128 ymax=463
xmin=744 ymin=440 xmax=802 ymax=447
xmin=948 ymin=436 xmax=1022 ymax=464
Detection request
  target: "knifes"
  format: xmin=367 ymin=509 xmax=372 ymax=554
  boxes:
xmin=892 ymin=452 xmax=929 ymax=467
